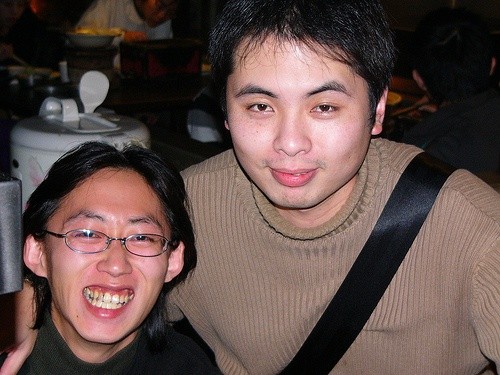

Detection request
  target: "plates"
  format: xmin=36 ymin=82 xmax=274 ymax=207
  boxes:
xmin=43 ymin=113 xmax=121 ymax=133
xmin=387 ymin=92 xmax=402 ymax=106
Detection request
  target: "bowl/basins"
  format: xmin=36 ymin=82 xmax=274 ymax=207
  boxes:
xmin=65 ymin=32 xmax=115 ymax=49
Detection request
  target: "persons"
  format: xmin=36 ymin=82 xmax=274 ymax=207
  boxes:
xmin=0 ymin=0 xmax=500 ymax=375
xmin=71 ymin=0 xmax=178 ymax=40
xmin=0 ymin=140 xmax=225 ymax=375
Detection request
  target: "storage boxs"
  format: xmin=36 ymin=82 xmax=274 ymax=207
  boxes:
xmin=120 ymin=38 xmax=206 ymax=74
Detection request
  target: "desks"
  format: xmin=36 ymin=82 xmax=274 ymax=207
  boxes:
xmin=0 ymin=66 xmax=207 ymax=111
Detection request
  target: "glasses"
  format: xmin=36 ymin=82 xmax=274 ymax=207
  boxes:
xmin=45 ymin=228 xmax=177 ymax=257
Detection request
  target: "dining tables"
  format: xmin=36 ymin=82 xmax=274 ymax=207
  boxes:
xmin=377 ymin=75 xmax=432 ymax=139
